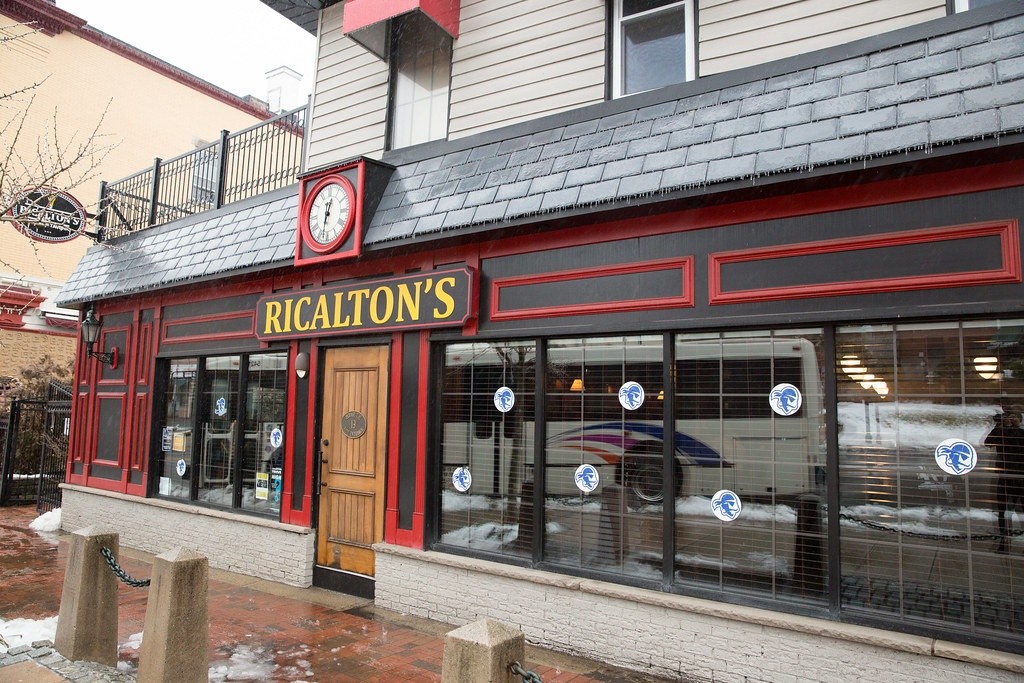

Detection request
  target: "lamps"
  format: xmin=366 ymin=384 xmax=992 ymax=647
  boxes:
xmin=80 ymin=302 xmax=119 ymax=370
xmin=294 ymin=350 xmax=310 ymax=378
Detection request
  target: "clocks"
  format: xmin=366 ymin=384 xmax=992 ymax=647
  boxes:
xmin=302 ymin=173 xmax=357 ymax=254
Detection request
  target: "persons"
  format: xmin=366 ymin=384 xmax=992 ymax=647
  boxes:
xmin=984 ymin=406 xmax=1024 ymax=555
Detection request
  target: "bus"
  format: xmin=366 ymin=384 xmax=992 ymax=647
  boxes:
xmin=162 ymin=336 xmax=821 ymax=509
